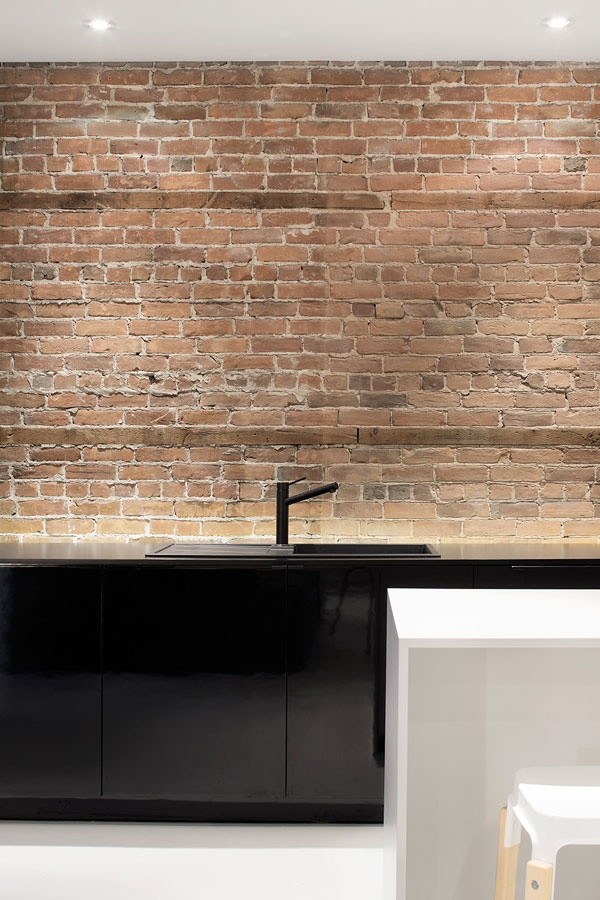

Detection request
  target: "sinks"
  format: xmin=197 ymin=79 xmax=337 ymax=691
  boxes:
xmin=289 ymin=542 xmax=443 ymax=560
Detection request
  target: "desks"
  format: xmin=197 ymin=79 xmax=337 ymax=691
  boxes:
xmin=383 ymin=587 xmax=600 ymax=898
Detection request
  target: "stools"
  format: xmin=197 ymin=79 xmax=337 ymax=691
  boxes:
xmin=494 ymin=784 xmax=600 ymax=899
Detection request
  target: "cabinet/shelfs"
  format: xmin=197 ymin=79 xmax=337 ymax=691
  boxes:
xmin=0 ymin=562 xmax=600 ymax=805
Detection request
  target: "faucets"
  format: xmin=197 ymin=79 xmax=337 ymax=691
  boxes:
xmin=275 ymin=476 xmax=340 ymax=549
